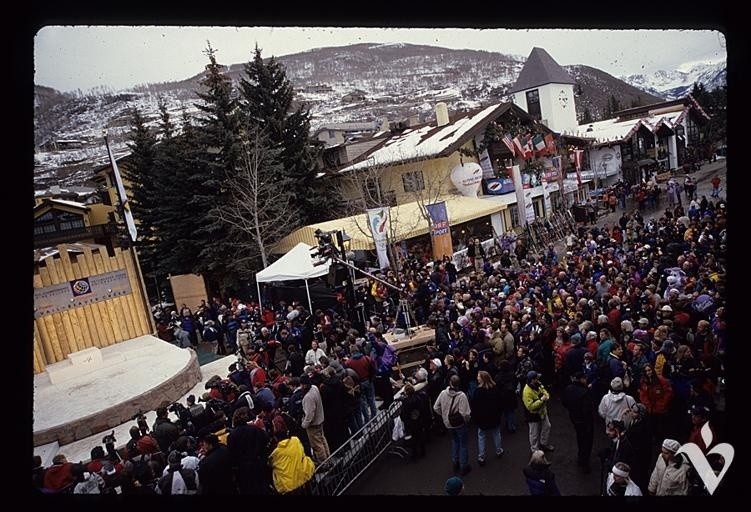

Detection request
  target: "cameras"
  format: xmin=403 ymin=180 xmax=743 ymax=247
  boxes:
xmin=169 ymin=402 xmax=184 ymax=413
xmin=132 ymin=409 xmax=149 ymax=435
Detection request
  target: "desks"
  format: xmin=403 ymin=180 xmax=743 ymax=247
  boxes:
xmin=382 ymin=324 xmax=436 ymax=371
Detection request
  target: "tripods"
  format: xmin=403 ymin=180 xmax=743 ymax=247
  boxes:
xmin=391 ymin=298 xmax=420 ymax=339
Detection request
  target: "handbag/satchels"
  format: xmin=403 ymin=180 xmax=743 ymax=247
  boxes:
xmin=448 ymin=412 xmax=464 ymax=427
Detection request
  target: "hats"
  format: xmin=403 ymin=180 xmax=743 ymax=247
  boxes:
xmin=444 ymin=477 xmax=462 ymax=495
xmin=688 ymin=405 xmax=710 ymax=417
xmin=662 ymin=438 xmax=681 ymax=452
xmin=527 ymin=371 xmax=541 ymax=379
xmin=610 ymin=245 xmax=680 ymax=392
xmin=430 ymin=358 xmax=442 ymax=366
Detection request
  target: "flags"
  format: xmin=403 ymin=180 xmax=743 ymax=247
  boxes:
xmin=503 ymin=132 xmax=556 ymax=159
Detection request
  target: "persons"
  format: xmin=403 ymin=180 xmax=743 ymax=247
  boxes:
xmin=34 ymin=168 xmax=726 ymax=496
